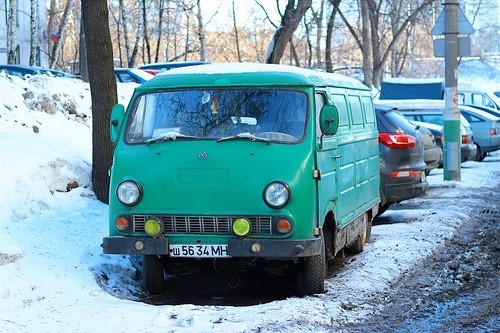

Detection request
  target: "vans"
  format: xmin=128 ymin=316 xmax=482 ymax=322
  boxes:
xmin=458 ymin=89 xmax=500 ymax=109
xmin=99 ymin=61 xmax=380 ymax=293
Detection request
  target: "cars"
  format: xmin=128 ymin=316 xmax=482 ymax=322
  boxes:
xmin=113 ymin=62 xmax=211 ymax=84
xmin=374 ymin=98 xmax=500 ymax=217
xmin=0 ymin=64 xmax=74 ymax=80
